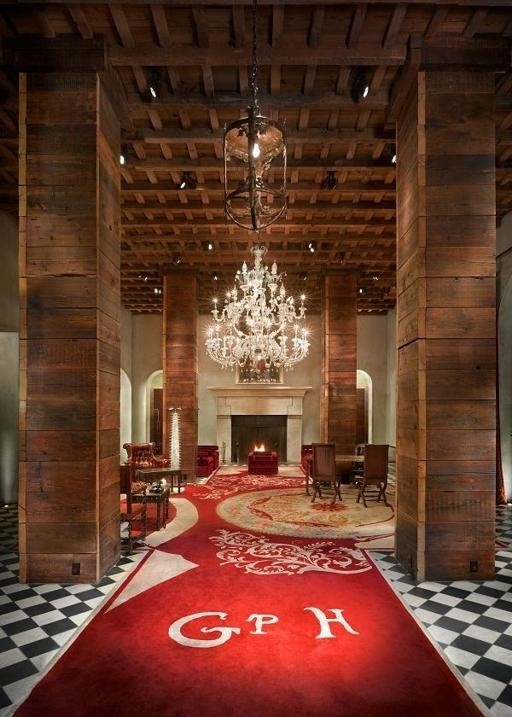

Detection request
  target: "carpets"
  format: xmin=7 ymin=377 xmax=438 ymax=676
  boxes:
xmin=6 ymin=470 xmax=494 ymax=716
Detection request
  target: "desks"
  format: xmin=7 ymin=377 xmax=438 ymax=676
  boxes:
xmin=132 ymin=483 xmax=171 ymax=531
xmin=305 ymin=454 xmax=364 ymax=500
xmin=137 ymin=467 xmax=182 ymax=494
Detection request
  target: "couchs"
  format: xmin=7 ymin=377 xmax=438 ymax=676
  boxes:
xmin=196 ymin=445 xmax=220 ymax=478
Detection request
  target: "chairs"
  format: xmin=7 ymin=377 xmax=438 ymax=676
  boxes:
xmin=310 ymin=442 xmax=343 ymax=505
xmin=123 ymin=441 xmax=169 ymax=482
xmin=354 ymin=443 xmax=390 ymax=508
xmin=119 ymin=464 xmax=147 ymax=552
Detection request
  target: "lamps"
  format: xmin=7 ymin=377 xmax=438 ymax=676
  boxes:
xmin=204 ymin=235 xmax=313 ymax=374
xmin=220 ymin=0 xmax=291 ymax=232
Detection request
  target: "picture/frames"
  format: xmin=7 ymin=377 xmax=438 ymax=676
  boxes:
xmin=234 ymin=319 xmax=285 ymax=387
